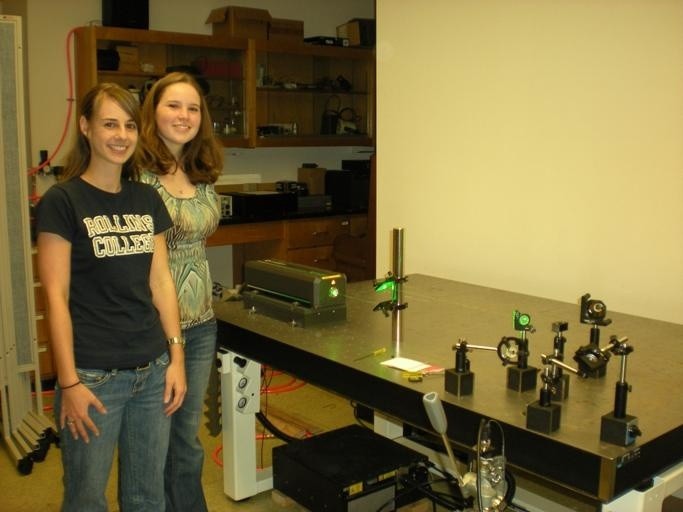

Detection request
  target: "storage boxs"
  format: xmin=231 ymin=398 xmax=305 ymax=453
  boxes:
xmin=203 ymin=5 xmax=304 ymax=46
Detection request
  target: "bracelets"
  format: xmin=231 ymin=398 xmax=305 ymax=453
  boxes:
xmin=167 ymin=336 xmax=186 ymax=349
xmin=60 ymin=380 xmax=82 ymax=391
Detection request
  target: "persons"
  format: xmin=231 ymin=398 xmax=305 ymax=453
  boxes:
xmin=33 ymin=83 xmax=187 ymax=511
xmin=132 ymin=70 xmax=224 ymax=511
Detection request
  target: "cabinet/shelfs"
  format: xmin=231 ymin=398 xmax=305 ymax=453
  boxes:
xmin=273 ymin=217 xmax=371 ymax=281
xmin=253 ymin=36 xmax=374 ymax=149
xmin=74 ymin=25 xmax=253 ymax=149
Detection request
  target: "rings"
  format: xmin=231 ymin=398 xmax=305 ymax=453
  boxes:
xmin=65 ymin=419 xmax=75 ymax=426
